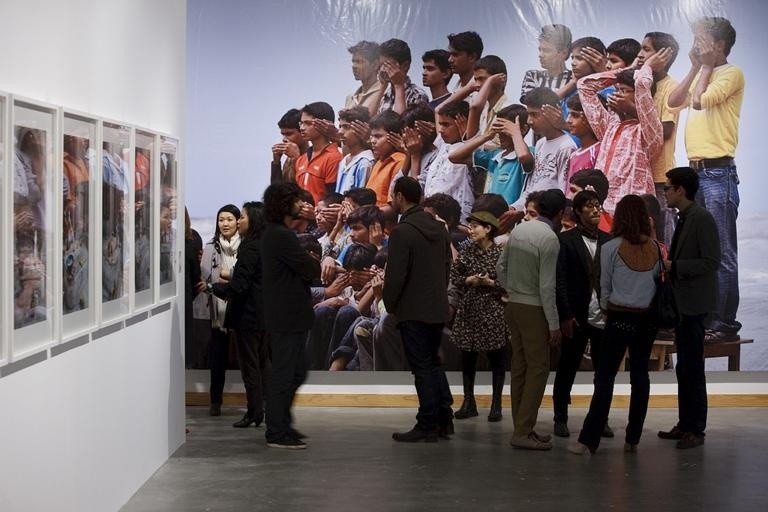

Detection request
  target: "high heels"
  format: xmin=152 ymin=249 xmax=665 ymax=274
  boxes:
xmin=232 ymin=408 xmax=265 ymax=428
xmin=567 ymin=442 xmax=588 ymax=454
xmin=624 ymin=443 xmax=638 ymax=450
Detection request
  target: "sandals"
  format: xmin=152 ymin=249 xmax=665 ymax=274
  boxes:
xmin=704 ymin=328 xmax=741 ymax=343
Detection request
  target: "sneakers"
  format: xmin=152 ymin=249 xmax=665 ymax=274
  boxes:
xmin=266 ymin=434 xmax=307 ymax=449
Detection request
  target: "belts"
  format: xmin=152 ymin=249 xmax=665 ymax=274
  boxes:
xmin=689 ymin=158 xmax=736 ymax=169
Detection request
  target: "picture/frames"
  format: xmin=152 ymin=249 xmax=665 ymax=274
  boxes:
xmin=185 ymin=0 xmax=768 ymax=383
xmin=132 ymin=126 xmax=156 ymax=312
xmin=157 ymin=133 xmax=179 ymax=314
xmin=8 ymin=92 xmax=57 ymax=359
xmin=100 ymin=116 xmax=131 ymax=320
xmin=60 ymin=105 xmax=101 ymax=339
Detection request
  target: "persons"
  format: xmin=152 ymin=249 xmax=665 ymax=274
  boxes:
xmin=542 ymin=37 xmax=606 ymax=129
xmin=382 ymin=177 xmax=454 ymax=442
xmin=364 ymin=108 xmax=406 ymax=206
xmin=455 ymin=56 xmax=513 ymax=144
xmin=200 ymin=205 xmax=244 ymax=415
xmin=291 ymin=102 xmax=341 ymax=201
xmin=448 ymin=104 xmax=535 ymax=213
xmin=258 ymin=183 xmax=321 ymax=450
xmin=495 ymin=189 xmax=566 ymax=451
xmin=270 ymin=109 xmax=312 ymax=184
xmin=554 ymin=191 xmax=617 ymax=439
xmin=565 ymin=93 xmax=608 ymax=193
xmin=392 ymin=102 xmax=438 ymax=200
xmin=421 ymin=50 xmax=454 ymax=116
xmin=658 ymin=167 xmax=720 ymax=450
xmin=424 ymin=74 xmax=508 ymax=220
xmin=447 ymin=31 xmax=483 ymax=98
xmin=577 ymin=48 xmax=673 ymax=237
xmin=498 ymin=87 xmax=577 ymax=235
xmin=608 ymin=32 xmax=680 ymax=194
xmin=184 ymin=207 xmax=204 ymax=368
xmin=336 ymin=106 xmax=373 ymax=195
xmin=668 ymin=17 xmax=745 ymax=344
xmin=12 ymin=108 xmax=176 ymax=326
xmin=567 ymin=195 xmax=662 ymax=455
xmin=604 ymin=39 xmax=642 ymax=72
xmin=301 ymin=191 xmax=399 ymax=371
xmin=520 ymin=24 xmax=604 ymax=107
xmin=569 ymin=170 xmax=614 ymax=232
xmin=342 ymin=41 xmax=380 ymax=116
xmin=196 ymin=201 xmax=267 ymax=428
xmin=450 ymin=212 xmax=509 ymax=421
xmin=376 ymin=39 xmax=425 ymax=116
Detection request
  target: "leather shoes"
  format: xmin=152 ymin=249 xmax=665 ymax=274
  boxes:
xmin=454 ymin=397 xmax=478 ymax=419
xmin=554 ymin=422 xmax=569 ymax=436
xmin=602 ymin=422 xmax=614 ymax=438
xmin=392 ymin=420 xmax=439 ymax=443
xmin=488 ymin=394 xmax=503 ymax=422
xmin=210 ymin=403 xmax=221 ymax=415
xmin=676 ymin=433 xmax=704 ymax=448
xmin=512 ymin=432 xmax=552 ymax=451
xmin=532 ymin=428 xmax=551 ymax=443
xmin=439 ymin=416 xmax=455 ymax=437
xmin=658 ymin=426 xmax=678 ymax=440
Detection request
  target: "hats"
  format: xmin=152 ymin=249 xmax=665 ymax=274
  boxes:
xmin=466 ymin=211 xmax=498 ymax=230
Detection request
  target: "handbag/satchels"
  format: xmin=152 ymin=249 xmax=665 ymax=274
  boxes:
xmin=649 ymin=239 xmax=678 ymax=329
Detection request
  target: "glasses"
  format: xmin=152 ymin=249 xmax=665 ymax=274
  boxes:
xmin=298 ymin=121 xmax=312 ymax=126
xmin=339 ymin=124 xmax=350 ymax=130
xmin=664 ymin=185 xmax=675 ymax=191
xmin=585 ymin=203 xmax=602 ymax=211
